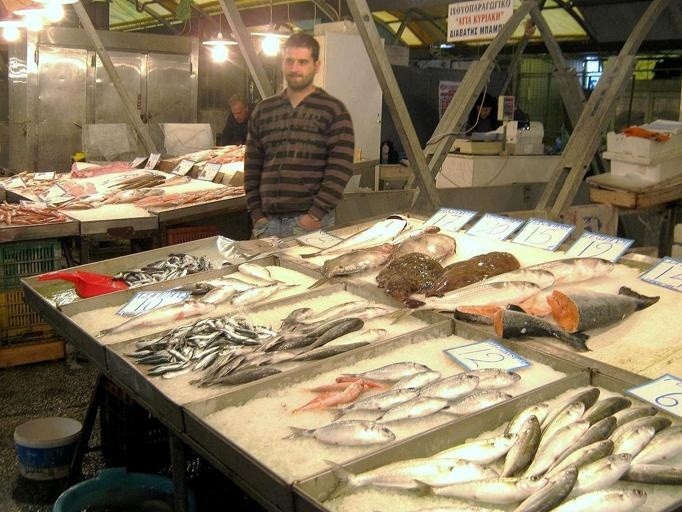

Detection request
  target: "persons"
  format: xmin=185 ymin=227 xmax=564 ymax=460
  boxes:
xmin=221 ymin=92 xmax=256 ymax=145
xmin=243 ymin=32 xmax=354 ymax=238
xmin=466 ymin=92 xmax=526 ymax=136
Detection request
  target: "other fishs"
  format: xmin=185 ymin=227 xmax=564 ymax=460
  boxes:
xmin=276 ymin=362 xmax=682 ymax=512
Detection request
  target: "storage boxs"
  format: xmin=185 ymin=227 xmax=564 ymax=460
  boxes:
xmin=602 ymin=119 xmax=682 ymax=183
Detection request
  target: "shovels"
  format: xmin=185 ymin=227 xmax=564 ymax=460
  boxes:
xmin=36 ymin=270 xmax=129 ymax=298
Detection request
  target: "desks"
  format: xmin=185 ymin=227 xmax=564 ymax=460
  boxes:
xmin=586 ymin=172 xmax=682 ymax=258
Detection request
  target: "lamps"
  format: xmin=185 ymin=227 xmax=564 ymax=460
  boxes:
xmin=12 ymin=1 xmax=50 ymax=33
xmin=0 ymin=10 xmax=26 ymax=44
xmin=385 ymin=8 xmax=456 ymax=67
xmin=202 ymin=9 xmax=239 ymax=64
xmin=261 ymin=21 xmax=295 ymax=57
xmin=31 ymin=0 xmax=80 ymax=22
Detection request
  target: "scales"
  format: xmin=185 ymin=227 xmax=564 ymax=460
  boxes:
xmin=449 ymin=96 xmax=515 ymax=155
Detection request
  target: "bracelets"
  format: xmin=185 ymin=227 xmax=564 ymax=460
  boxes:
xmin=310 ymin=214 xmax=319 ymax=221
